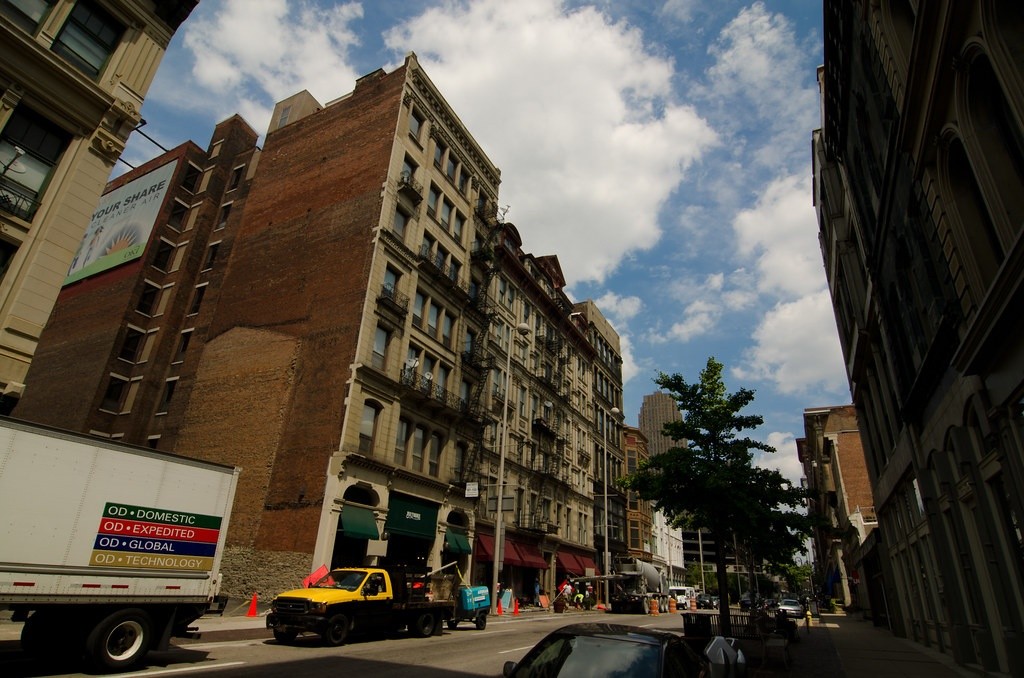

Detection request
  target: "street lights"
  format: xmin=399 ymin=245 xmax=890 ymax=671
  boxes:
xmin=604 ymin=407 xmax=619 ymax=609
xmin=489 ymin=323 xmax=530 ymax=616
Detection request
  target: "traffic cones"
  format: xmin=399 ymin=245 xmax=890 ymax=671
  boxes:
xmin=245 ymin=592 xmax=258 ymax=617
xmin=597 ymin=604 xmax=608 ymax=610
xmin=512 ymin=598 xmax=521 ymax=615
xmin=497 ymin=600 xmax=503 ymax=614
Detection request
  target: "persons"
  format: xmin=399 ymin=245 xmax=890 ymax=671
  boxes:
xmin=532 ymin=577 xmax=539 ymax=605
xmin=563 ymin=582 xmax=573 ymax=603
xmin=574 ymin=594 xmax=585 ymax=608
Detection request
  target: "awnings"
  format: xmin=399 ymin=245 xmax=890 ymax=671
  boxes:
xmin=445 ymin=533 xmax=472 ymax=554
xmin=342 ymin=506 xmax=381 ymax=540
xmin=475 ymin=535 xmax=600 ymax=574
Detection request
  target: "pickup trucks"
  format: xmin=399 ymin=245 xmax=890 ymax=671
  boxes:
xmin=266 ymin=561 xmax=491 ymax=647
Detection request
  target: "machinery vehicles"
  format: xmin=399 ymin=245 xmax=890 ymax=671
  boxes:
xmin=608 ymin=553 xmax=720 ymax=614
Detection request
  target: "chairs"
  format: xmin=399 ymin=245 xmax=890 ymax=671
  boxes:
xmin=755 ymin=616 xmax=794 ymax=671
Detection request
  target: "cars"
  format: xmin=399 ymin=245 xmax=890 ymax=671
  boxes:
xmin=503 ymin=622 xmax=711 ymax=678
xmin=739 ymin=592 xmax=805 ymax=619
